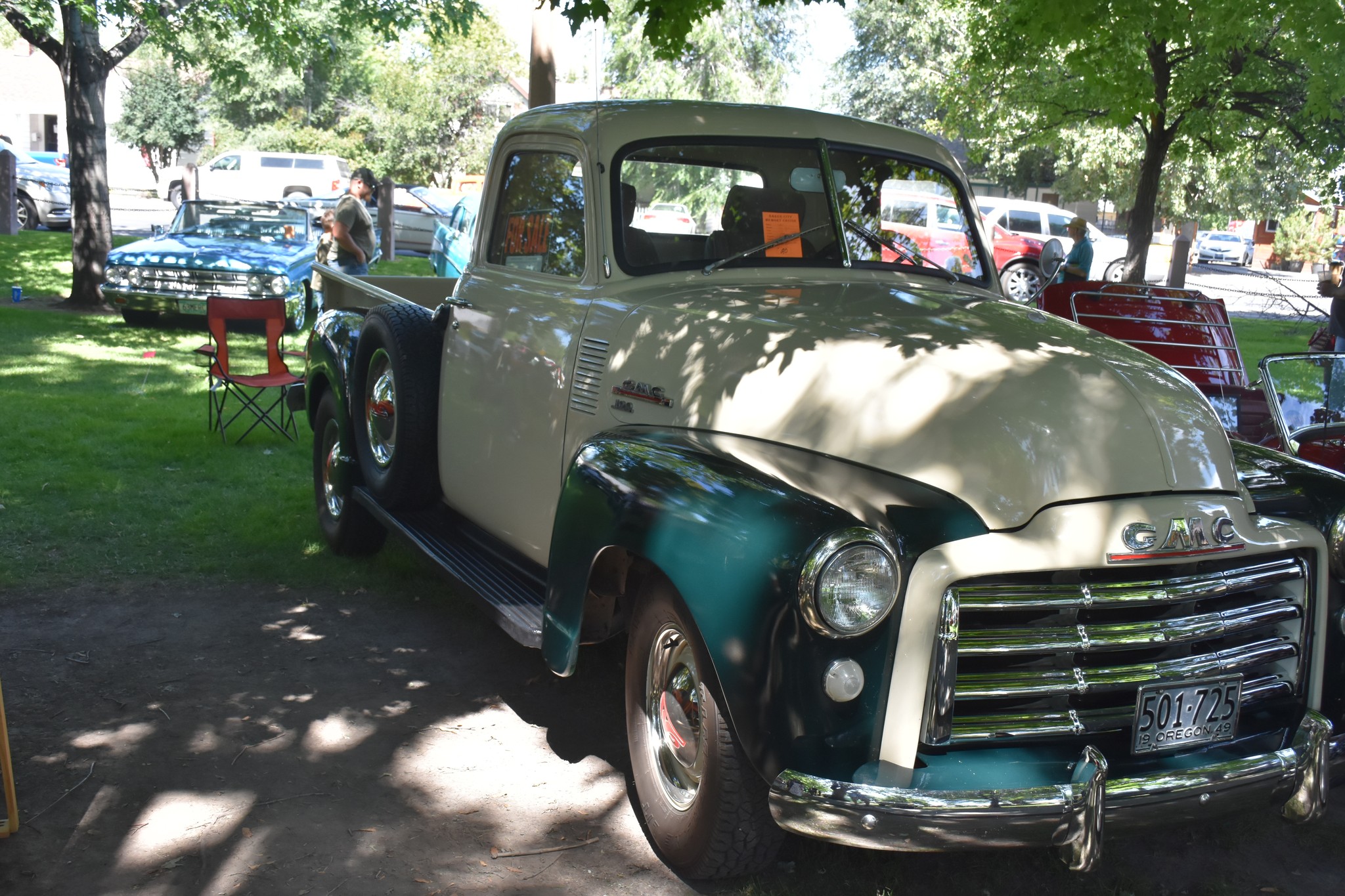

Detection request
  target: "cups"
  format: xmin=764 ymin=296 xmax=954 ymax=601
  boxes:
xmin=1317 ymin=271 xmax=1333 ymax=284
xmin=1328 ymin=262 xmax=1342 ymax=286
xmin=12 ymin=286 xmax=22 ymax=304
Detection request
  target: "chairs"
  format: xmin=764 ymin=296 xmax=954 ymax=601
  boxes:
xmin=191 ymin=293 xmax=307 ymax=449
xmin=698 ymin=180 xmax=818 ymax=266
xmin=562 ymin=176 xmax=660 ymax=278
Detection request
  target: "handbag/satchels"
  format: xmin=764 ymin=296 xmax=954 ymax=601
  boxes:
xmin=1307 ymin=327 xmax=1334 ymax=353
xmin=1063 ymin=260 xmax=1087 ymax=282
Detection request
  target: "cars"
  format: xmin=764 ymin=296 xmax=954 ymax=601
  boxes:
xmin=98 ymin=199 xmax=326 ymax=335
xmin=367 ymin=175 xmax=488 ymax=254
xmin=949 ymin=194 xmax=1164 ymax=285
xmin=1036 ymin=281 xmax=1345 ymax=469
xmin=643 ymin=202 xmax=698 ymax=235
xmin=306 ymin=102 xmax=1345 ymax=888
xmin=1198 ymin=230 xmax=1255 ymax=267
xmin=429 ymin=196 xmax=481 ymax=280
xmin=0 ymin=137 xmax=73 ymax=231
xmin=868 ymin=188 xmax=1068 ymax=304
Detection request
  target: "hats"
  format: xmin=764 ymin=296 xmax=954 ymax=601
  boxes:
xmin=352 ymin=167 xmax=381 ymax=184
xmin=1063 ymin=217 xmax=1091 ymax=232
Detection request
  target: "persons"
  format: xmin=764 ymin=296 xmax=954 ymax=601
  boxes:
xmin=1316 ymin=266 xmax=1345 ymax=409
xmin=326 ymin=167 xmax=376 ymax=276
xmin=310 ymin=208 xmax=337 ymax=319
xmin=1056 ymin=217 xmax=1094 ymax=284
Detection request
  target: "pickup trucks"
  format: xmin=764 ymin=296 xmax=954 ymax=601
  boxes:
xmin=24 ymin=151 xmax=70 ymax=168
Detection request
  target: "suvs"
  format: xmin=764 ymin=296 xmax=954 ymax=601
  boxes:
xmin=155 ymin=152 xmax=353 ymax=214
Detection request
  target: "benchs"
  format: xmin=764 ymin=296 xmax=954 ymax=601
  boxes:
xmin=1195 ymin=381 xmax=1277 ymax=444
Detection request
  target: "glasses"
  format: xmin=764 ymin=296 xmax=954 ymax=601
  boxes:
xmin=362 ymin=180 xmax=379 ymax=190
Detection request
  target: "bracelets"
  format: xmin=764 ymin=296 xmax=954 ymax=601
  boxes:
xmin=1064 ymin=265 xmax=1067 ymax=272
